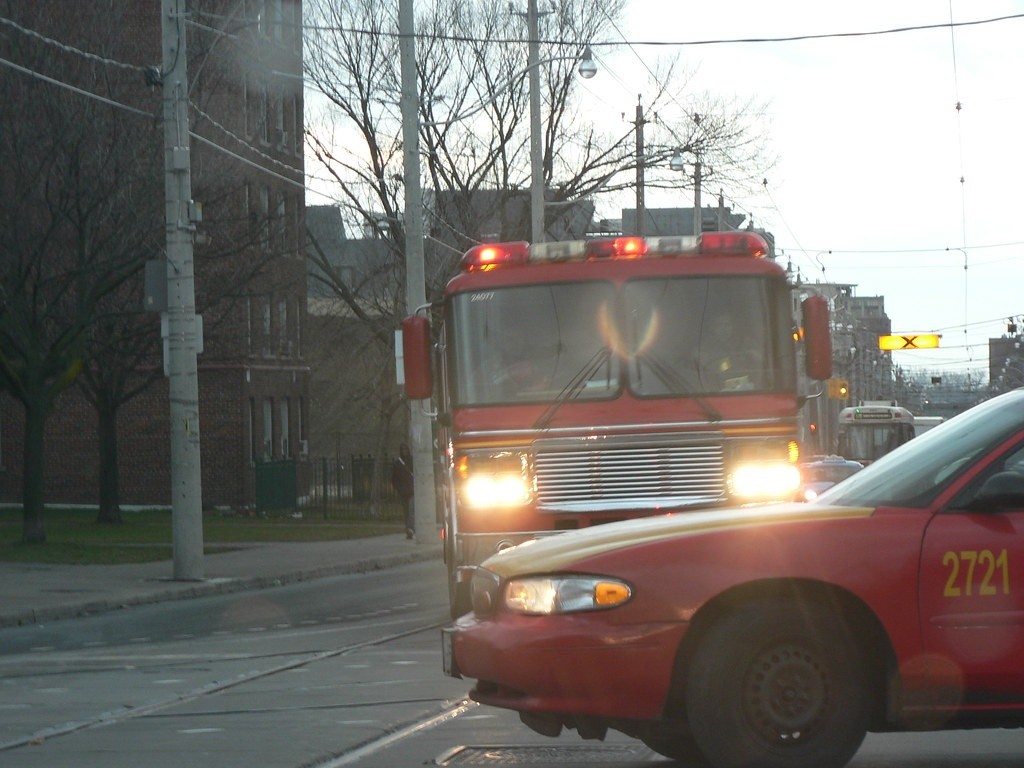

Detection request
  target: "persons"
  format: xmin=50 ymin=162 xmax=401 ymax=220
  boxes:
xmin=393 ymin=444 xmax=416 ymax=539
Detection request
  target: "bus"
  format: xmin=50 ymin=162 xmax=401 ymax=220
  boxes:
xmin=834 ymin=399 xmax=916 ymax=470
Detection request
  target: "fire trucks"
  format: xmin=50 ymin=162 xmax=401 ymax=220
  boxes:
xmin=399 ymin=230 xmax=837 ymax=692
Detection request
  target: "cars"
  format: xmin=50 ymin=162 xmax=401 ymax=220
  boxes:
xmin=440 ymin=385 xmax=1024 ymax=768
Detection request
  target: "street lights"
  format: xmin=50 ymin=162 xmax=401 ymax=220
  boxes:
xmin=399 ymin=46 xmax=603 ymax=547
xmin=530 ymin=144 xmax=684 ymax=248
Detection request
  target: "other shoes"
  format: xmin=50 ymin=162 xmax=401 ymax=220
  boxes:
xmin=407 ymin=529 xmax=413 ymax=540
